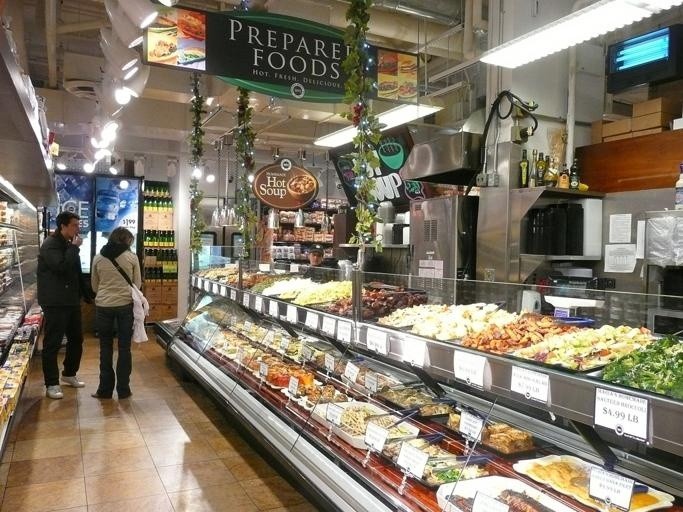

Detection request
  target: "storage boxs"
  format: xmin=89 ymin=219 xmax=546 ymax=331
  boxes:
xmin=600 ymin=96 xmax=678 ymax=145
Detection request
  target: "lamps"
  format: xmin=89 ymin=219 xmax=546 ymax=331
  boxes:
xmin=54 ymin=152 xmax=344 ymax=190
xmin=313 ymin=96 xmax=444 ymax=150
xmin=228 ymin=128 xmax=244 ymax=227
xmin=221 ymin=133 xmax=231 ymax=227
xmin=268 ymin=147 xmax=278 ymax=159
xmin=81 ymin=0 xmax=176 ymax=177
xmin=297 ymin=147 xmax=306 ymax=160
xmin=209 ymin=138 xmax=220 ymax=229
xmin=478 ymin=1 xmax=682 ymax=70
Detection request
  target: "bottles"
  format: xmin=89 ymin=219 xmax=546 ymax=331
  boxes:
xmin=672 ymin=162 xmax=682 ymax=211
xmin=144 ymin=188 xmax=179 ymax=277
xmin=519 ymin=147 xmax=588 ymax=190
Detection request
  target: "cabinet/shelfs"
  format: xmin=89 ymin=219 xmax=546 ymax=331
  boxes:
xmin=508 ymin=183 xmax=605 ymax=289
xmin=0 ymin=173 xmax=42 ymax=473
xmin=141 ymin=178 xmax=176 ymax=325
xmin=258 ymin=196 xmax=346 ymax=267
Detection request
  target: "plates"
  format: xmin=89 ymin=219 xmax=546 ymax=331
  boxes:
xmin=186 ymin=265 xmax=683 ymax=511
xmin=379 ymin=60 xmax=418 ymax=99
xmin=149 ymin=22 xmax=206 ymax=64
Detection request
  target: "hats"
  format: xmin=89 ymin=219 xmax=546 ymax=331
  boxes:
xmin=309 ymin=243 xmax=324 ymax=255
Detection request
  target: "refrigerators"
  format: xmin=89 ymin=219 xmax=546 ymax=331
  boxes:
xmin=45 ymin=171 xmax=143 ymax=272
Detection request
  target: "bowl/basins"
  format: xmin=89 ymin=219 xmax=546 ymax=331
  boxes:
xmin=288 ymin=175 xmax=316 ymax=196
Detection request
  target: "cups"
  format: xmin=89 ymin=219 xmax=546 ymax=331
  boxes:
xmin=377 ymin=143 xmax=406 ymax=171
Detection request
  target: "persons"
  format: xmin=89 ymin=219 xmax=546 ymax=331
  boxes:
xmin=91 ymin=229 xmax=142 ymax=400
xmin=301 ymin=244 xmax=335 ymax=285
xmin=38 ymin=211 xmax=96 ymax=400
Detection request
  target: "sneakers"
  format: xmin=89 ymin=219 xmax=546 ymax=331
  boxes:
xmin=117 ymin=391 xmax=133 ymax=400
xmin=61 ymin=373 xmax=85 ymax=388
xmin=91 ymin=392 xmax=113 ymax=399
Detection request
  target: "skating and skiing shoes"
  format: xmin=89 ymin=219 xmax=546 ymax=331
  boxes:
xmin=45 ymin=384 xmax=64 ymax=399
